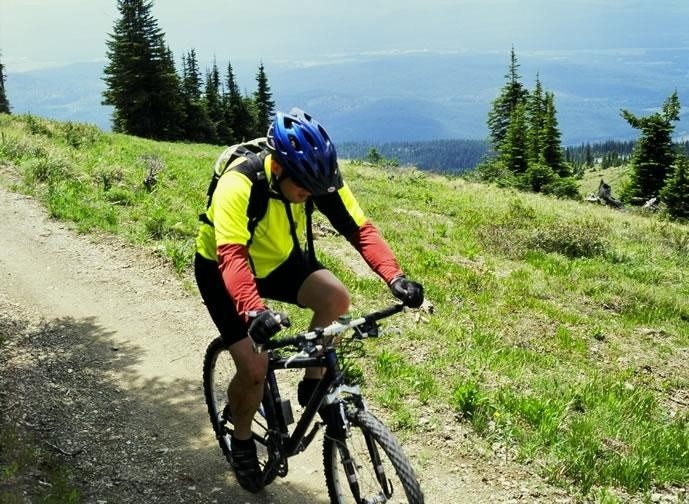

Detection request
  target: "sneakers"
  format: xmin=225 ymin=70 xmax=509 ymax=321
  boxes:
xmin=228 ymin=431 xmax=265 ymax=494
xmin=296 ymin=378 xmax=351 ymax=407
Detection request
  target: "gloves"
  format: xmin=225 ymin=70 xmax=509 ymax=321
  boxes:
xmin=249 ymin=310 xmax=289 ymax=346
xmin=389 ymin=278 xmax=426 ymax=312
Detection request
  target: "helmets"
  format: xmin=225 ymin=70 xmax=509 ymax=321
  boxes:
xmin=265 ymin=106 xmax=341 ymax=194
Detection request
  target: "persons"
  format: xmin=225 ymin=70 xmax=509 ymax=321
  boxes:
xmin=193 ymin=106 xmax=424 ymax=494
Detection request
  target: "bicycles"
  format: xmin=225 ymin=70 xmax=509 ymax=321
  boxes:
xmin=199 ymin=292 xmax=432 ymax=503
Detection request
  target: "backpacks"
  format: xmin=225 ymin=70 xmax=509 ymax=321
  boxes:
xmin=204 ymin=135 xmax=271 ymax=240
